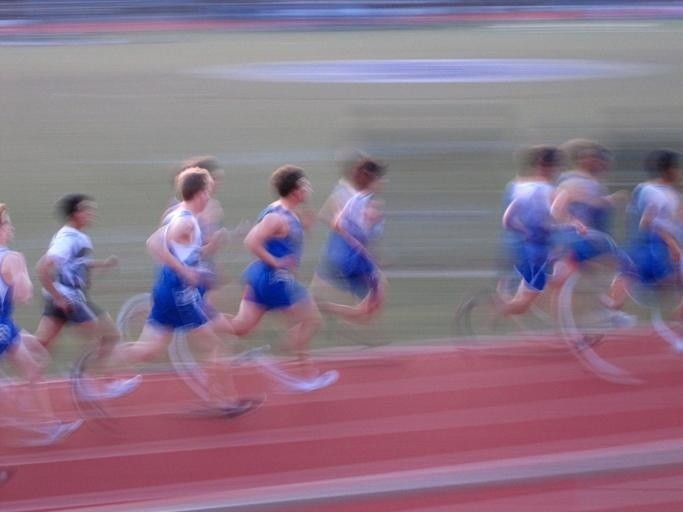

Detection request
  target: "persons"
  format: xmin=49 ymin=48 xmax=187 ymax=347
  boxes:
xmin=456 ymin=141 xmax=683 ymax=383
xmin=0 ymin=155 xmax=389 ymax=447
